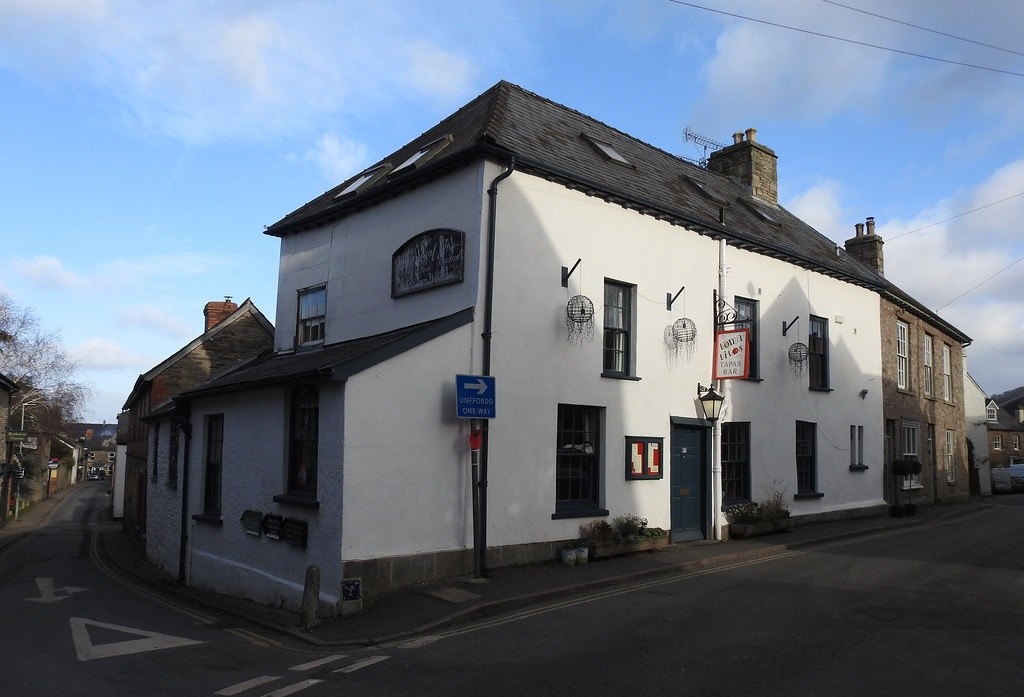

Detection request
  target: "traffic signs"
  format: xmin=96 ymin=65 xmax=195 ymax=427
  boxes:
xmin=454 ymin=373 xmax=497 ymax=418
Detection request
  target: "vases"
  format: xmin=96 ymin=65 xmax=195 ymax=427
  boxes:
xmin=728 ymin=517 xmax=794 ymax=541
xmin=580 ymin=530 xmax=669 ymax=559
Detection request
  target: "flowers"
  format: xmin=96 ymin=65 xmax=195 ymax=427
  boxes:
xmin=579 ymin=512 xmax=669 ymax=546
xmin=724 ymin=479 xmax=791 ymax=524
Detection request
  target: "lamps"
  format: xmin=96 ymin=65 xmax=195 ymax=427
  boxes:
xmin=562 ymin=258 xmax=595 ymax=348
xmin=667 ymin=285 xmax=697 ymax=360
xmin=697 ymin=381 xmax=726 ymax=428
xmin=784 ymin=316 xmax=809 ymax=379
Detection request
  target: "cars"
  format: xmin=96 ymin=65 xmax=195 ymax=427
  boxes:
xmin=88 ymin=472 xmax=99 ymax=480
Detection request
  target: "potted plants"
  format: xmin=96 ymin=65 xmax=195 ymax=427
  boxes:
xmin=561 ymin=543 xmax=578 ymax=566
xmin=576 ymin=541 xmax=589 ymax=564
xmin=893 ymin=455 xmax=923 ymax=517
